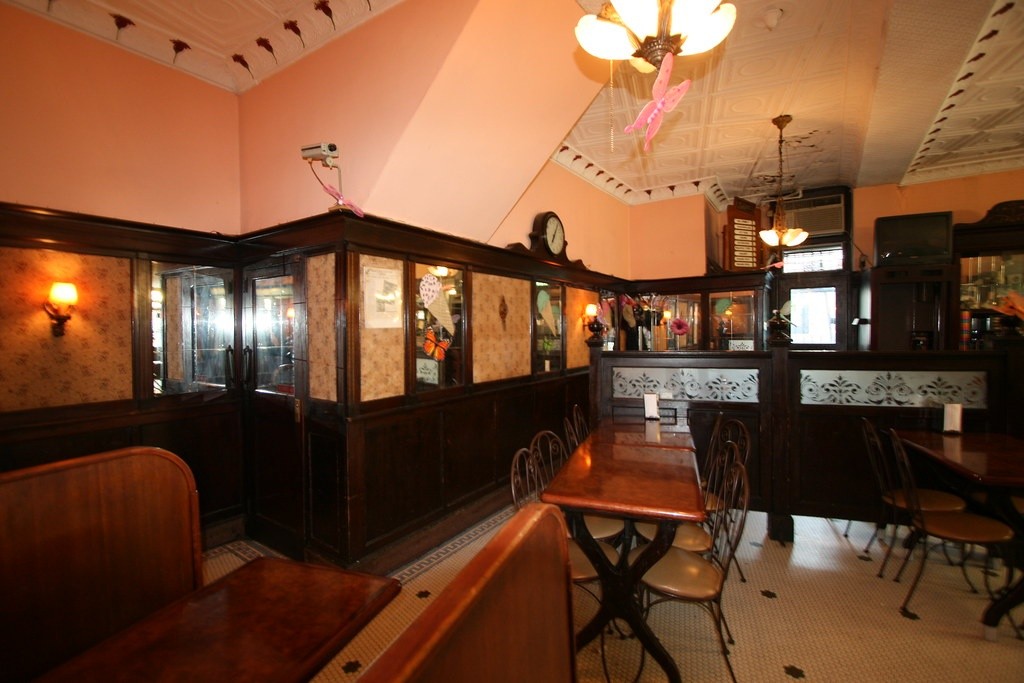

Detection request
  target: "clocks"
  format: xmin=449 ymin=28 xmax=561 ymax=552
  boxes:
xmin=540 ymin=211 xmax=566 ymax=259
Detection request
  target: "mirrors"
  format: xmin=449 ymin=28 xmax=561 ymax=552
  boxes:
xmin=151 ymin=259 xmax=234 ymax=398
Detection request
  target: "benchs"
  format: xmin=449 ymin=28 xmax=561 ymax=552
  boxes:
xmin=354 ymin=502 xmax=582 ymax=683
xmin=0 ymin=445 xmax=206 ymax=683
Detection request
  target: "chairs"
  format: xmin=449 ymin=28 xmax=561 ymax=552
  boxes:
xmin=702 ymin=416 xmax=751 ymax=584
xmin=627 ymin=460 xmax=749 ymax=683
xmin=527 ymin=429 xmax=626 ymax=633
xmin=629 ymin=440 xmax=742 ymax=644
xmin=887 ymin=427 xmax=1020 ymax=636
xmin=699 ymin=409 xmax=725 ymax=486
xmin=843 ymin=416 xmax=965 ymax=567
xmin=572 ymin=403 xmax=590 ymax=443
xmin=562 ymin=417 xmax=579 ymax=455
xmin=511 ymin=446 xmax=627 ymax=682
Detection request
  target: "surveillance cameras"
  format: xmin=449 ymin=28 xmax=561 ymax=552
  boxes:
xmin=301 ymin=143 xmax=339 ymax=160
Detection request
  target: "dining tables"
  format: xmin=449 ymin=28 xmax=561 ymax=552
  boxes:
xmin=878 ymin=428 xmax=1024 ymax=642
xmin=540 ymin=442 xmax=708 ymax=683
xmin=583 ymin=415 xmax=697 ymax=453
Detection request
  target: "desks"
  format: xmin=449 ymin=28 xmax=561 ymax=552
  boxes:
xmin=39 ymin=551 xmax=404 ymax=683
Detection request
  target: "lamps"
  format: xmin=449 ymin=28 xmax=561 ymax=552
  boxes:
xmin=43 ymin=282 xmax=78 ymax=336
xmin=759 ymin=114 xmax=810 ymax=247
xmin=573 ymin=0 xmax=737 ymax=74
